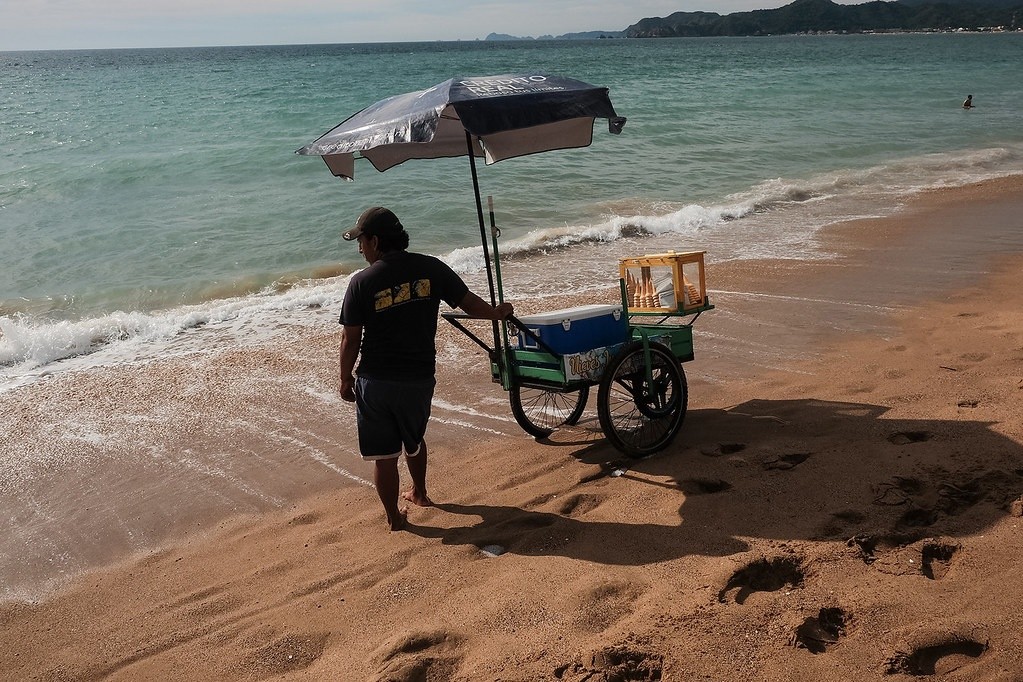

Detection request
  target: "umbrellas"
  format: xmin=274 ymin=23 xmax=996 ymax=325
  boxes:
xmin=294 ymin=75 xmax=625 ymax=351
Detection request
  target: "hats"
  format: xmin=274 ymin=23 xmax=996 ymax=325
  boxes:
xmin=341 ymin=207 xmax=399 ymax=241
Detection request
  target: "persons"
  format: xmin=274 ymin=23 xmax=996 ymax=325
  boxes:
xmin=339 ymin=206 xmax=514 ymax=529
xmin=963 ymin=94 xmax=972 ymax=106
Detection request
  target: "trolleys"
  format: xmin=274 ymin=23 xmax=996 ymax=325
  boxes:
xmin=441 ymin=277 xmax=716 ymax=460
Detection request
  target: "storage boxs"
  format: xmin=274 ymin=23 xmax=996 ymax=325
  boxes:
xmin=515 ymin=304 xmax=628 ymax=355
